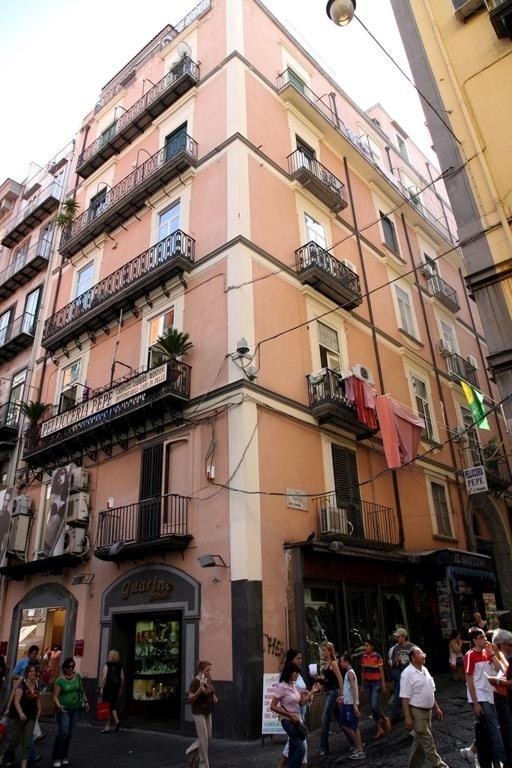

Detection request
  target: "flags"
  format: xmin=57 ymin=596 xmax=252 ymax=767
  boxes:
xmin=460 ymin=379 xmax=491 ymax=431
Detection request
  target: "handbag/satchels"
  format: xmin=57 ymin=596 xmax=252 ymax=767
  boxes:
xmin=97 ymin=694 xmax=110 ymax=720
xmin=457 ymin=657 xmax=462 ymax=666
xmin=292 ymin=719 xmax=309 ymax=741
xmin=78 ymin=678 xmax=83 ymax=705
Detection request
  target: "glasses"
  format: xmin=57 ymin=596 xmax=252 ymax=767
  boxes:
xmin=66 ymin=664 xmax=74 ymax=668
xmin=417 ymin=653 xmax=426 ymax=657
xmin=475 ymin=635 xmax=488 ymax=639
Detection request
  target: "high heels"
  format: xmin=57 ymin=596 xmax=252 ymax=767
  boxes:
xmin=113 ymin=721 xmax=125 ymax=733
xmin=99 ymin=728 xmax=113 ymax=737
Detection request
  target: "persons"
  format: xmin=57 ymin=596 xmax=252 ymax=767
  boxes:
xmin=0 ymin=645 xmax=41 ymax=768
xmin=99 ymin=650 xmax=125 ymax=735
xmin=42 ymin=645 xmax=62 ymax=689
xmin=269 ymin=612 xmax=512 ymax=768
xmin=51 ymin=657 xmax=90 ymax=767
xmin=184 ymin=660 xmax=218 ymax=768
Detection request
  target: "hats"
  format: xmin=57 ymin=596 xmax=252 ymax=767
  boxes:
xmin=392 ymin=628 xmax=408 ymax=637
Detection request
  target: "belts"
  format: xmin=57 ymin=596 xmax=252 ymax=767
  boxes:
xmin=409 ymin=704 xmax=430 ymax=712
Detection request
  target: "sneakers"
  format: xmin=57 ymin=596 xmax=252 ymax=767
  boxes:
xmin=345 ymin=751 xmax=367 ymax=760
xmin=6 ymin=762 xmax=16 ymax=768
xmin=343 ymin=748 xmax=358 ymax=759
xmin=33 ymin=732 xmax=70 ymax=767
xmin=459 ymin=747 xmax=477 ymax=768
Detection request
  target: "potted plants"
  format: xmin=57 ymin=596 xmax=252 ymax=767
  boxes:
xmin=146 ymin=325 xmax=194 ymax=394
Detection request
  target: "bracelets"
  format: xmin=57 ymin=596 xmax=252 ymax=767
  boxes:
xmin=84 ymin=701 xmax=89 ymax=704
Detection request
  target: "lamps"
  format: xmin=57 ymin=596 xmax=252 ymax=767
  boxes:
xmin=196 ymin=552 xmax=231 ymax=569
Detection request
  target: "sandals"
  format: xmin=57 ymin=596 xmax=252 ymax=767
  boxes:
xmin=372 ymin=728 xmax=386 ymax=738
xmin=385 ymin=716 xmax=393 ymax=737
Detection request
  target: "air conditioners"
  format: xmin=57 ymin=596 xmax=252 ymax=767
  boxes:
xmin=6 ymin=465 xmax=94 ymax=559
xmin=466 ymin=354 xmax=478 ymax=372
xmin=318 ymin=167 xmax=342 ymax=196
xmin=352 ymin=361 xmax=374 ymax=385
xmin=419 ymin=259 xmax=435 ymax=278
xmin=437 ymin=336 xmax=452 ymax=354
xmin=314 ymin=502 xmax=354 ymax=540
xmin=338 ymin=257 xmax=358 ymax=279
